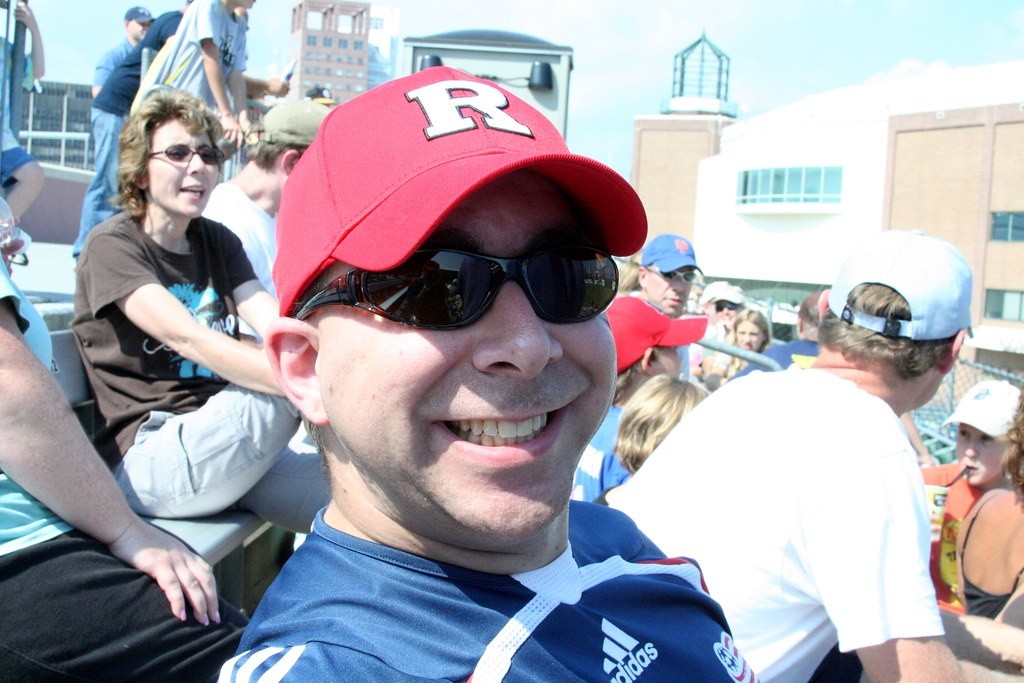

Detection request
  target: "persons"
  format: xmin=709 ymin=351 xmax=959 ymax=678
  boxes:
xmin=571 ymin=295 xmax=707 ymax=504
xmin=0 ymin=0 xmax=337 ymax=683
xmin=614 ymin=229 xmax=1024 ymax=683
xmin=215 ymin=65 xmax=758 ymax=681
xmin=406 ymin=255 xmax=488 ymax=326
xmin=578 ymin=251 xmax=615 ymax=318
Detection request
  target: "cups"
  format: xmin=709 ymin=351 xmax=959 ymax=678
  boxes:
xmin=923 ymin=484 xmax=950 ymax=543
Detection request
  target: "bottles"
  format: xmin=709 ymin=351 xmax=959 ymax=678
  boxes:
xmin=0 ymin=195 xmax=32 ymax=254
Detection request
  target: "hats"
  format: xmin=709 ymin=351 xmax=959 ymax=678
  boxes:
xmin=124 ymin=7 xmax=156 ymax=22
xmin=273 ymin=66 xmax=647 ymax=316
xmin=258 ymin=100 xmax=333 ymax=145
xmin=828 ymin=231 xmax=974 ymax=340
xmin=604 ymin=298 xmax=707 ymax=371
xmin=700 ymin=281 xmax=731 ymax=304
xmin=713 ymin=291 xmax=744 ymax=304
xmin=942 ymin=379 xmax=1021 ymax=436
xmin=642 ymin=235 xmax=702 ymax=275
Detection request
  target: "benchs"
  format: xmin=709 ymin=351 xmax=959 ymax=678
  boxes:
xmin=47 ymin=328 xmax=267 ymax=623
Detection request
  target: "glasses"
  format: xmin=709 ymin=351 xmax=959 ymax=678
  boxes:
xmin=296 ymin=244 xmax=618 ymax=329
xmin=149 ymin=145 xmax=225 ymax=165
xmin=647 ymin=266 xmax=696 ymax=282
xmin=716 ymin=302 xmax=737 ymax=310
